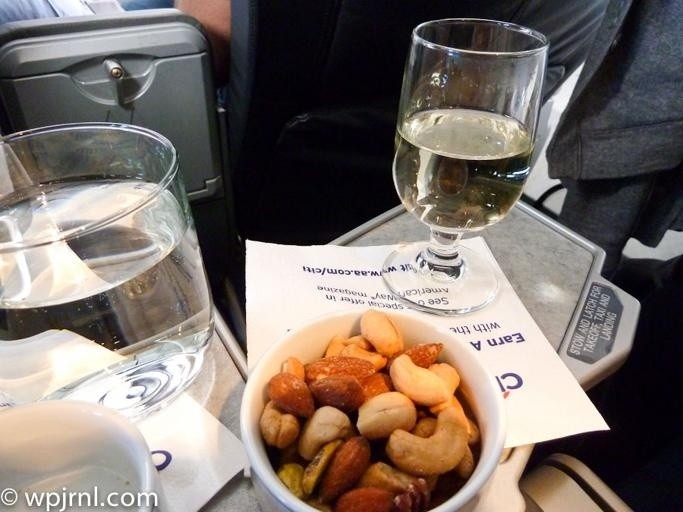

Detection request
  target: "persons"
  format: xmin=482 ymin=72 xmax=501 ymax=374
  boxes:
xmin=175 ymin=0 xmax=231 ymax=49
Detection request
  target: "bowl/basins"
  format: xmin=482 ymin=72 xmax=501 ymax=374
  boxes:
xmin=240 ymin=308 xmax=509 ymax=512
xmin=0 ymin=399 xmax=167 ymax=512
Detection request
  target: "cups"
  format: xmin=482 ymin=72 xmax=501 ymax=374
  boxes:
xmin=0 ymin=122 xmax=215 ymax=425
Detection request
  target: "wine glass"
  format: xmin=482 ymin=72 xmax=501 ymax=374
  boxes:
xmin=381 ymin=18 xmax=550 ymax=317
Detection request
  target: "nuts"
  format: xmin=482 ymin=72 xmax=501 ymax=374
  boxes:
xmin=259 ymin=309 xmax=480 ymax=512
